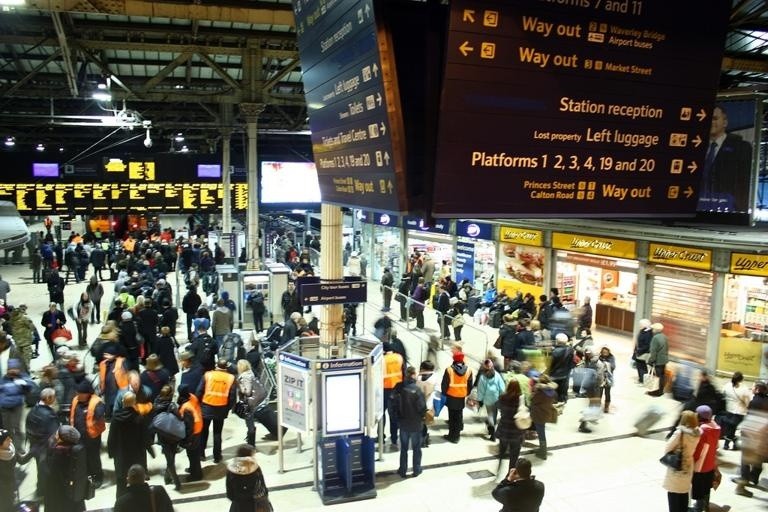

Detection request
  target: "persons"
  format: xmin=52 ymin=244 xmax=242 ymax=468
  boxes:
xmin=645 ymin=322 xmax=668 ymax=397
xmin=685 ymin=404 xmax=720 ymax=512
xmin=1 ymin=219 xmax=616 ymax=512
xmin=731 ymin=379 xmax=768 ymax=486
xmin=682 ymin=370 xmax=710 ymax=412
xmin=698 ymin=383 xmax=722 ymax=416
xmin=632 ymin=319 xmax=651 ymax=384
xmin=695 ymin=106 xmax=751 ymax=211
xmin=662 ymin=409 xmax=704 ymax=512
xmin=734 ymin=397 xmax=768 ymax=497
xmin=721 ymin=371 xmax=753 ymax=451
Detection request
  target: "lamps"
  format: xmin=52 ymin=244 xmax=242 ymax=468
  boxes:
xmin=91 ymin=75 xmax=112 ymax=101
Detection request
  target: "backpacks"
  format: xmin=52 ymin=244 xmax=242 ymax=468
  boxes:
xmin=218 ymin=332 xmax=242 ymax=364
xmin=197 ymin=337 xmax=216 ymax=366
xmin=388 ymin=382 xmax=411 ymax=425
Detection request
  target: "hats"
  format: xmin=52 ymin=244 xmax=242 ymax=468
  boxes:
xmin=290 ymin=312 xmax=302 ymax=319
xmin=696 ymin=405 xmax=712 ymax=419
xmin=555 ymin=333 xmax=568 ymax=344
xmin=0 ymin=357 xmax=94 ymax=445
xmin=421 ymin=351 xmax=493 ymax=370
xmin=102 ymin=310 xmax=133 ymax=357
xmin=640 ymin=319 xmax=650 ymax=328
xmin=651 ymin=322 xmax=664 ymax=333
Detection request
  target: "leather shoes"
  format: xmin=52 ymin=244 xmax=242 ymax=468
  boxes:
xmin=398 ymin=470 xmax=406 ymax=479
xmin=214 ymin=455 xmax=222 ymax=463
xmin=413 ymin=470 xmax=422 ymax=477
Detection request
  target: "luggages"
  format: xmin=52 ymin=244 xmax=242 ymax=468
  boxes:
xmin=254 ymin=403 xmax=288 ymax=439
xmin=633 ymin=399 xmax=685 ymax=435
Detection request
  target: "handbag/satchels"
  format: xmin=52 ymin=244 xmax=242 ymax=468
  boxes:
xmin=660 ymin=446 xmax=684 ymax=472
xmin=52 ymin=309 xmax=103 ymax=357
xmin=150 ymin=402 xmax=186 ymax=442
xmin=513 ymin=395 xmax=533 ymax=431
xmin=234 ymin=376 xmax=267 ymax=419
xmin=543 ymin=405 xmax=559 ymax=423
xmin=709 ymin=468 xmax=722 ymax=491
xmin=493 ymin=334 xmax=503 ymax=349
xmin=432 ymin=391 xmax=447 ymax=418
xmin=643 ymin=365 xmax=660 ymax=392
xmin=87 ymin=474 xmax=95 ymax=501
xmin=451 ymin=313 xmax=467 ymax=329
xmin=254 ymin=485 xmax=274 ymax=512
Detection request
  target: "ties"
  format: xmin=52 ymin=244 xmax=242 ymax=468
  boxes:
xmin=707 ymin=140 xmax=717 ymax=164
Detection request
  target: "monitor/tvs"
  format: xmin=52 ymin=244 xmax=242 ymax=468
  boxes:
xmin=258 ymin=151 xmax=321 ymax=214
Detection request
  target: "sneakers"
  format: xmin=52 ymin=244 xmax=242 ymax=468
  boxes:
xmin=163 ymin=455 xmax=206 ymax=491
xmin=444 ymin=434 xmax=459 ymax=443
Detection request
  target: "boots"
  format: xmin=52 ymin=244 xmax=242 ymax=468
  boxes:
xmin=537 ymin=441 xmax=546 ymax=460
xmin=724 ymin=438 xmax=739 ymax=450
xmin=734 ymin=478 xmax=756 ymax=498
xmin=486 ymin=423 xmax=497 ymax=442
xmin=605 ymin=400 xmax=611 ymax=413
